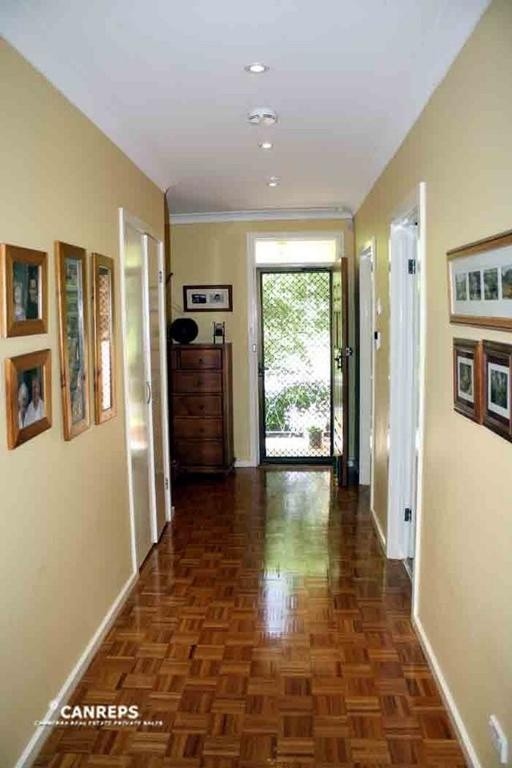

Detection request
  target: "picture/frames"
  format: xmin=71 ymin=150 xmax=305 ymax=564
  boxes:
xmin=452 ymin=337 xmax=482 ymax=426
xmin=183 ymin=285 xmax=233 ymax=312
xmin=1 ymin=243 xmax=49 ymax=340
xmin=5 ymin=348 xmax=53 ymax=450
xmin=53 ymin=240 xmax=93 ymax=441
xmin=481 ymin=339 xmax=512 ymax=443
xmin=445 ymin=228 xmax=511 ymax=334
xmin=89 ymin=254 xmax=118 ymax=425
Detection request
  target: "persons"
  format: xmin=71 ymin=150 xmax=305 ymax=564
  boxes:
xmin=12 ymin=274 xmax=42 ymax=322
xmin=15 ymin=374 xmax=48 ymax=429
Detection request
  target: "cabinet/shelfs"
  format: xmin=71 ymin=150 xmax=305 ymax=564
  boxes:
xmin=171 ymin=343 xmax=236 ymax=476
